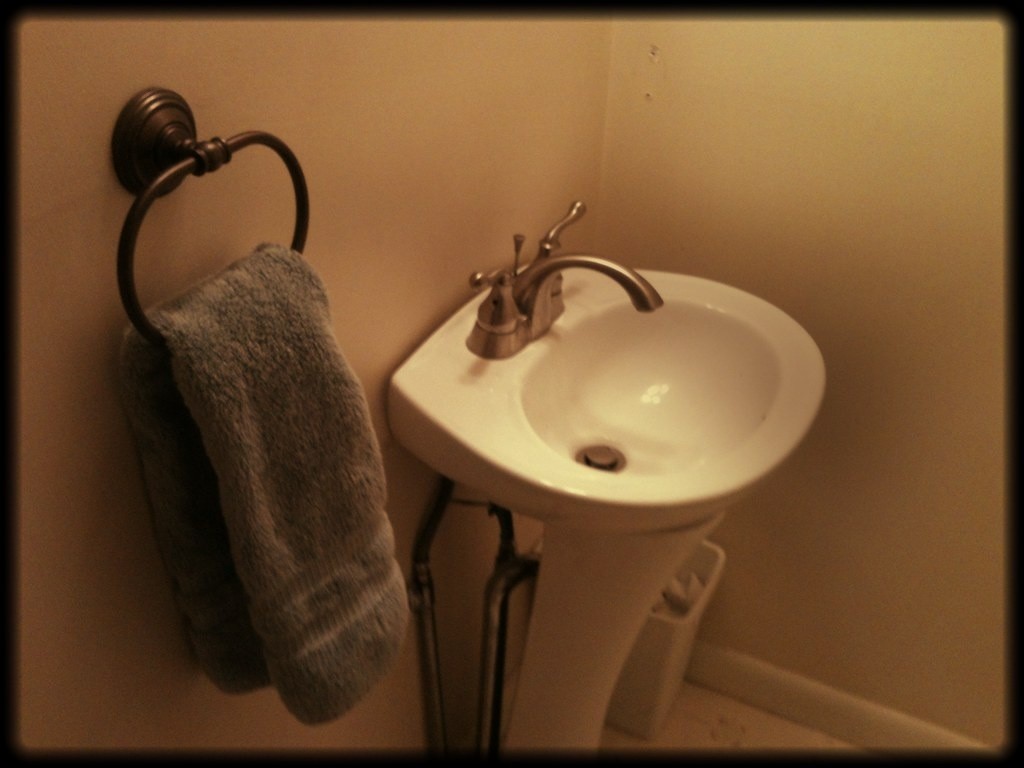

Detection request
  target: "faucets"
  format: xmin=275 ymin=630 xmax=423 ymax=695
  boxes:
xmin=512 ymin=253 xmax=664 ymax=315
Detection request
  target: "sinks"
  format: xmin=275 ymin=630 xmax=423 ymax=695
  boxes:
xmin=385 ymin=260 xmax=832 ymax=534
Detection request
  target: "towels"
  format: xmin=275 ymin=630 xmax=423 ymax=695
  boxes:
xmin=116 ymin=236 xmax=415 ymax=730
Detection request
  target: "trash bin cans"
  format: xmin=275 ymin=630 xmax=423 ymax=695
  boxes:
xmin=603 ymin=538 xmax=728 ymax=741
